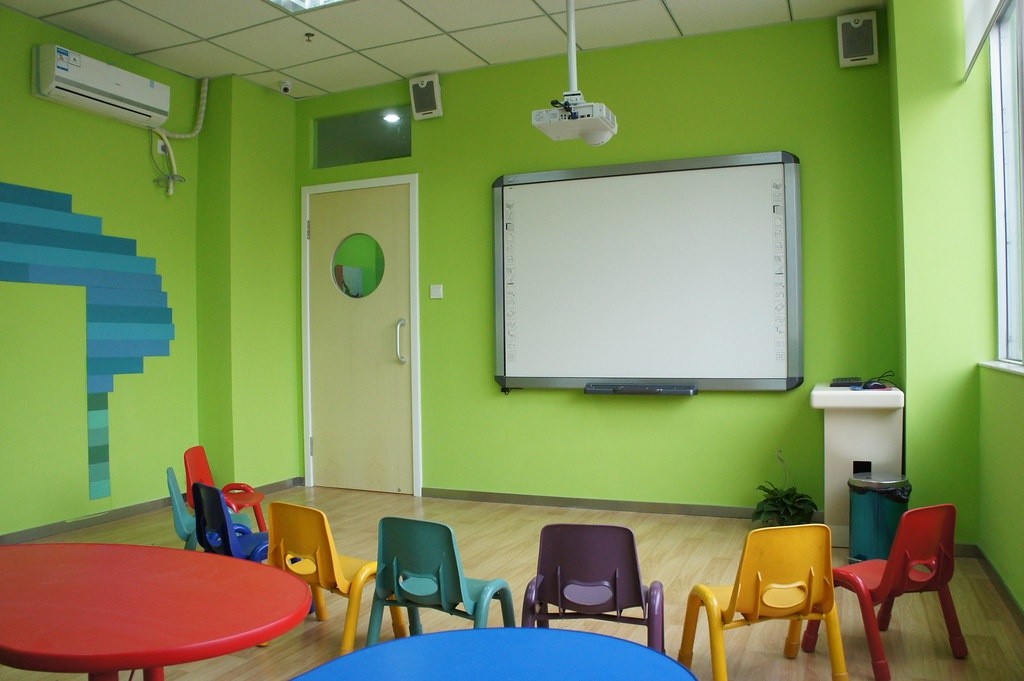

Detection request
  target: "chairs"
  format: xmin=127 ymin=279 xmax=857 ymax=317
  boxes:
xmin=801 ymin=503 xmax=970 ymax=681
xmin=521 ymin=524 xmax=666 ymax=657
xmin=366 ymin=516 xmax=513 ymax=647
xmin=192 ymin=482 xmax=315 ymax=614
xmin=678 ymin=524 xmax=849 ymax=681
xmin=166 ymin=466 xmax=253 ymax=551
xmin=184 ymin=446 xmax=267 ymax=532
xmin=257 ymin=501 xmax=408 ymax=657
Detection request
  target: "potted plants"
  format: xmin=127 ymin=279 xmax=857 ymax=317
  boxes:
xmin=751 ymin=450 xmax=822 ymax=526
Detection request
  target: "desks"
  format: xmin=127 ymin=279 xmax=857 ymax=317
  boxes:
xmin=0 ymin=544 xmax=312 ymax=681
xmin=291 ymin=626 xmax=697 ymax=681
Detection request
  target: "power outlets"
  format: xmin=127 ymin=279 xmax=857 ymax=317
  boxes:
xmin=157 ymin=139 xmax=169 ymax=156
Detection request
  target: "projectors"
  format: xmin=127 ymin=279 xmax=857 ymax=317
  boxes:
xmin=532 ymin=102 xmax=617 ymax=145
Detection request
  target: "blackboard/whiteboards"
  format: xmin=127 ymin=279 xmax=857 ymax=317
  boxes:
xmin=491 ymin=149 xmax=804 ymax=395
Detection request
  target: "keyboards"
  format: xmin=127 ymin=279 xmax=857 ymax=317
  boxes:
xmin=830 ymin=377 xmax=863 ymax=388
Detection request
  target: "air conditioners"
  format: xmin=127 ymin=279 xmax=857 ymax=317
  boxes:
xmin=30 ymin=44 xmax=170 ymax=130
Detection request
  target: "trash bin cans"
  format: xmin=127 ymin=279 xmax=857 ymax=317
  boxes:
xmin=847 ymin=472 xmax=912 ymax=565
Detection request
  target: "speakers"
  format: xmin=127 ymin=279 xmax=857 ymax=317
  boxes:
xmin=837 ymin=10 xmax=878 ymax=68
xmin=409 ymin=73 xmax=443 ymax=121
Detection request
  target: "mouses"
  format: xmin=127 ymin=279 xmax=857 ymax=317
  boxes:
xmin=862 ymin=381 xmax=886 ymax=389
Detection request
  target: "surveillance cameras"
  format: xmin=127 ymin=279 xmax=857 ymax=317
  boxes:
xmin=279 ymin=82 xmax=291 ymax=94
